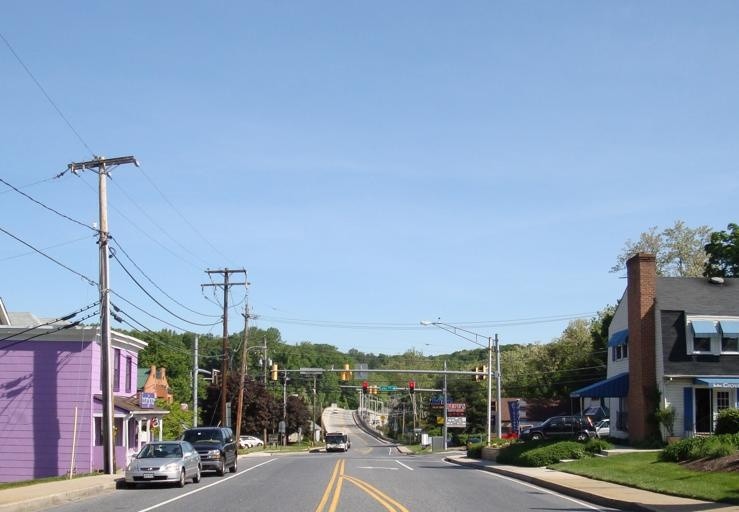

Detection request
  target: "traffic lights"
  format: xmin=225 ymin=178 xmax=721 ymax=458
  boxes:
xmin=217 ymin=371 xmax=223 ymax=390
xmin=471 ymin=366 xmax=478 ymax=381
xmin=363 ymin=382 xmax=367 ymax=393
xmin=479 ymin=364 xmax=486 ymax=379
xmin=410 ymin=381 xmax=415 ymax=393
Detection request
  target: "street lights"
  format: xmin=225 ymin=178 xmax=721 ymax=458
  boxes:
xmin=419 ymin=319 xmax=502 ymax=441
xmin=235 ymin=346 xmax=267 ymax=438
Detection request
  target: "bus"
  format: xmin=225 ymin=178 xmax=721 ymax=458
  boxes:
xmin=323 ymin=430 xmax=350 ymax=452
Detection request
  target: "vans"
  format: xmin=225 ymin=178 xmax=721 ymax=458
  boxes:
xmin=177 ymin=426 xmax=239 ymax=476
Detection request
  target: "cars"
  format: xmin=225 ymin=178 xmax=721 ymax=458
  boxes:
xmin=124 ymin=440 xmax=202 ymax=488
xmin=236 ymin=434 xmax=263 ymax=448
xmin=594 ymin=418 xmax=610 ymax=438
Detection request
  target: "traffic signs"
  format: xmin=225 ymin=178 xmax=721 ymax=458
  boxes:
xmin=380 ymin=386 xmax=398 ymax=391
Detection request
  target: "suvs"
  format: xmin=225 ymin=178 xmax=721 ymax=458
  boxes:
xmin=520 ymin=415 xmax=596 ymax=443
